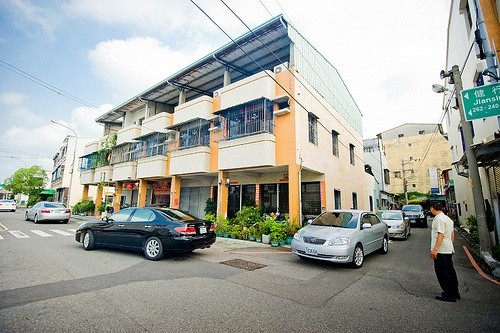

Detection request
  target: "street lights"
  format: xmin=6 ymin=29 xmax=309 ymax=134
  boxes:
xmin=51 ymin=119 xmax=78 ymax=208
xmin=11 ymin=156 xmax=26 ymax=194
xmin=431 ymin=83 xmax=492 ymax=252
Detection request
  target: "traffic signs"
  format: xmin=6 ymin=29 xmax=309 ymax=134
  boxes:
xmin=459 ymin=81 xmax=500 ymax=122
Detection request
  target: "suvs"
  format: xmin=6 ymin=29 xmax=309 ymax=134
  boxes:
xmin=401 ymin=205 xmax=428 ymax=227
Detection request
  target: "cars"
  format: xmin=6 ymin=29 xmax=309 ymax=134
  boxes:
xmin=75 ymin=206 xmax=217 ymax=261
xmin=376 ymin=209 xmax=411 ymax=241
xmin=0 ymin=200 xmax=17 ymax=212
xmin=25 ymin=201 xmax=71 ymax=223
xmin=291 ymin=210 xmax=390 ymax=269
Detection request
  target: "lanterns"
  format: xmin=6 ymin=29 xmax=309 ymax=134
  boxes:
xmin=127 ymin=183 xmax=134 ymax=189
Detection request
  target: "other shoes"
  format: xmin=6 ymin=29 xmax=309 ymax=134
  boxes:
xmin=436 ymin=295 xmax=461 ymax=302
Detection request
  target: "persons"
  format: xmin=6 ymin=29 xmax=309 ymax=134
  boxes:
xmin=428 ymin=200 xmax=461 ymax=303
